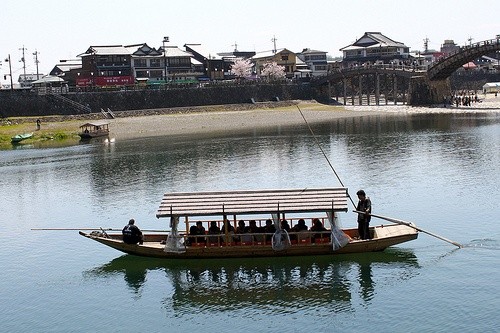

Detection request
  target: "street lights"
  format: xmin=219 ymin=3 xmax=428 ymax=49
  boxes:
xmin=162 ymin=40 xmax=170 ymax=80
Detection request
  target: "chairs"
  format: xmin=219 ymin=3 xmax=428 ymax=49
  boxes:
xmin=192 ymin=238 xmax=329 ymax=248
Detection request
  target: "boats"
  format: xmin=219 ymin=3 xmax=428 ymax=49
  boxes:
xmin=11 ymin=132 xmax=34 ymax=141
xmin=77 ymin=186 xmax=419 ymax=258
xmin=75 ymin=120 xmax=110 ymax=139
xmin=18 ymin=135 xmax=53 ymax=145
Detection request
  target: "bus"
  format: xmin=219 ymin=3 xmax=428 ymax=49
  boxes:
xmin=77 ymin=65 xmax=134 ymax=92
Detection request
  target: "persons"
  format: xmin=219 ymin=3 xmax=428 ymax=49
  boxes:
xmin=190 ymin=218 xmax=329 ymax=243
xmin=122 ymin=219 xmax=144 ymax=245
xmin=36 ymin=118 xmax=40 ymax=130
xmin=356 ymin=190 xmax=372 ymax=239
